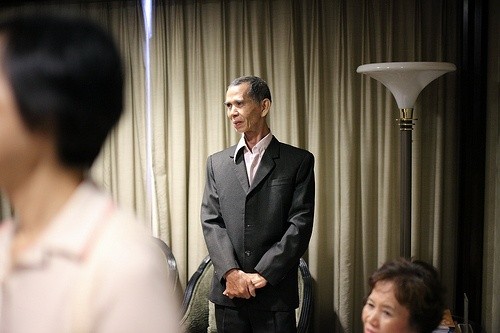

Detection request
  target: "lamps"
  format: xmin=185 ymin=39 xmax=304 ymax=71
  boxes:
xmin=357 ymin=62 xmax=456 ymax=264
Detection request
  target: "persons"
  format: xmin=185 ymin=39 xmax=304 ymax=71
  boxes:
xmin=0 ymin=6 xmax=190 ymax=333
xmin=200 ymin=76 xmax=317 ymax=333
xmin=360 ymin=255 xmax=444 ymax=333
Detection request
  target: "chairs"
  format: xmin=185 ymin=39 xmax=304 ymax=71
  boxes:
xmin=176 ymin=253 xmax=312 ymax=333
